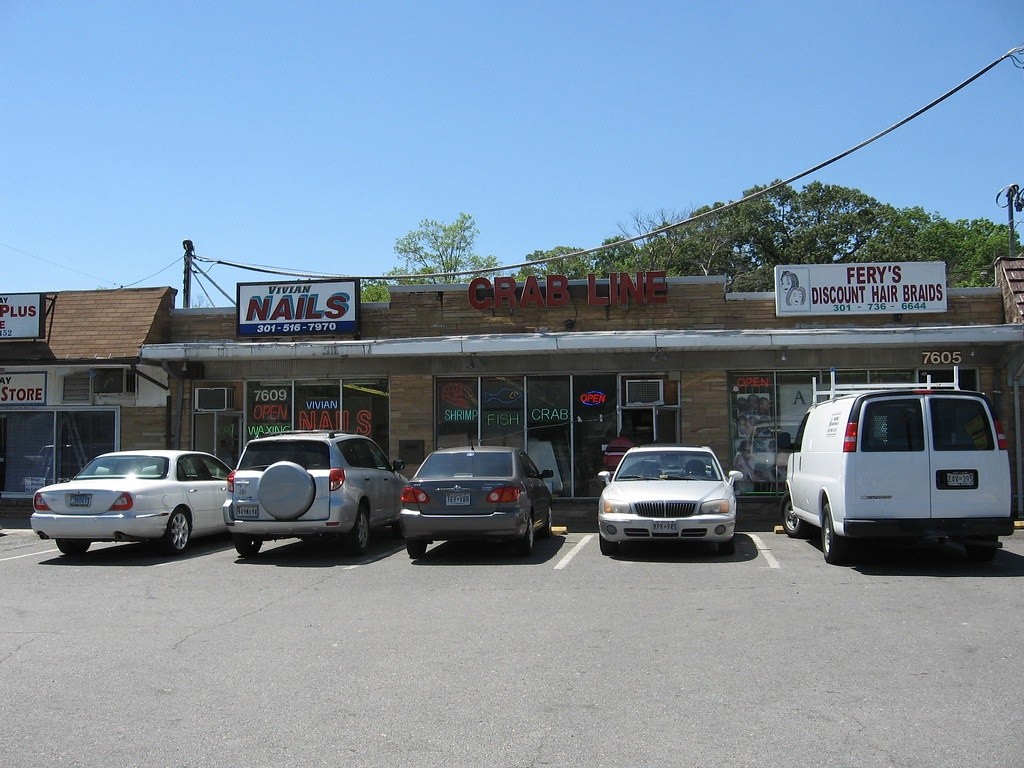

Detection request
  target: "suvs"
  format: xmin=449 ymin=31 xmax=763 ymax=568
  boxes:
xmin=223 ymin=428 xmax=416 ymax=557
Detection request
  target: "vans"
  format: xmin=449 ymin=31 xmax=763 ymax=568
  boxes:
xmin=777 ymin=392 xmax=1014 ymax=566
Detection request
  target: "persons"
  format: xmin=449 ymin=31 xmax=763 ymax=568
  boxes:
xmin=738 ymin=393 xmax=771 ymax=437
xmin=602 ymin=427 xmax=636 ymax=479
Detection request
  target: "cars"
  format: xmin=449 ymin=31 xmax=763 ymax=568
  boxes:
xmin=401 ymin=440 xmax=555 ymax=556
xmin=596 ymin=443 xmax=745 ymax=556
xmin=30 ymin=446 xmax=245 ymax=555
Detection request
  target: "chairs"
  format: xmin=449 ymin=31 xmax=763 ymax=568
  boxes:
xmin=636 ymin=459 xmax=663 ymax=478
xmin=94 ymin=466 xmax=110 ymax=476
xmin=141 ymin=464 xmax=162 ymax=476
xmin=682 ymin=460 xmax=709 ymax=478
